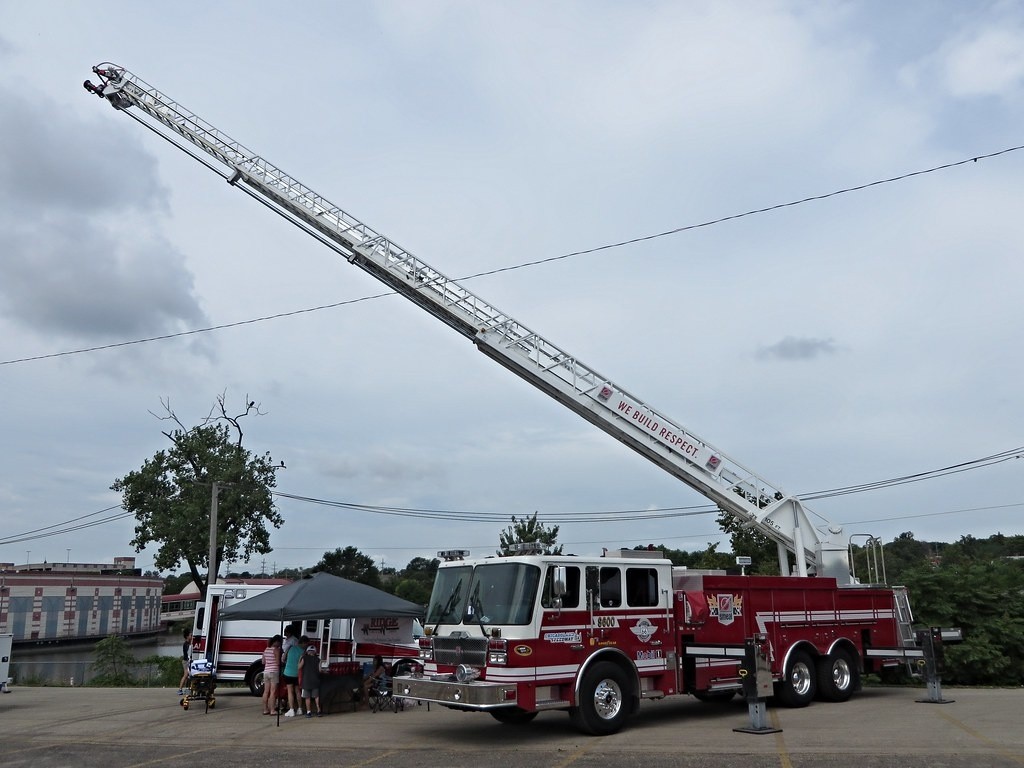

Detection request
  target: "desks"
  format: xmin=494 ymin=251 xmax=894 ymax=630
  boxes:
xmin=295 ymin=672 xmax=362 ymax=714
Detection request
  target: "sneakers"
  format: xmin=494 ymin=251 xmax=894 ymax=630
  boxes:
xmin=284 ymin=708 xmax=295 ymax=717
xmin=296 ymin=708 xmax=303 ymax=715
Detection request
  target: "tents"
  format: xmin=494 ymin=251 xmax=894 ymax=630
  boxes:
xmin=214 ymin=571 xmax=430 ymax=726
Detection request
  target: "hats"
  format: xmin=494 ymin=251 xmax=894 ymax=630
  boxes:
xmin=306 ymin=645 xmax=317 ymax=652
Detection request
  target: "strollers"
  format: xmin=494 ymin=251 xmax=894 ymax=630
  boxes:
xmin=181 ymin=658 xmax=217 ymax=710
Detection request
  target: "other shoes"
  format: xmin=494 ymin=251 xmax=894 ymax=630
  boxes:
xmin=316 ymin=711 xmax=322 ymax=716
xmin=270 ymin=710 xmax=282 ymax=716
xmin=263 ymin=711 xmax=270 ymax=715
xmin=305 ymin=712 xmax=312 ymax=718
xmin=177 ymin=688 xmax=183 ymax=695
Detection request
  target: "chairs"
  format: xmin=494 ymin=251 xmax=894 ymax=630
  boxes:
xmin=362 ymin=662 xmax=425 ymax=713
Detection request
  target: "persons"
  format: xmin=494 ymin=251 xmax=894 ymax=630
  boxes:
xmin=262 ymin=624 xmax=386 ymax=718
xmin=176 ymin=628 xmax=192 ymax=695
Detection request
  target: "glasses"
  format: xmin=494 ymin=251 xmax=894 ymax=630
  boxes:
xmin=189 ymin=635 xmax=192 ymax=637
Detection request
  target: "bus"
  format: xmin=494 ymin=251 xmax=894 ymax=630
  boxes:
xmin=190 ymin=583 xmax=423 ymax=696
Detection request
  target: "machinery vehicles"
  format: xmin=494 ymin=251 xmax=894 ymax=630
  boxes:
xmin=84 ymin=62 xmax=924 ymax=736
